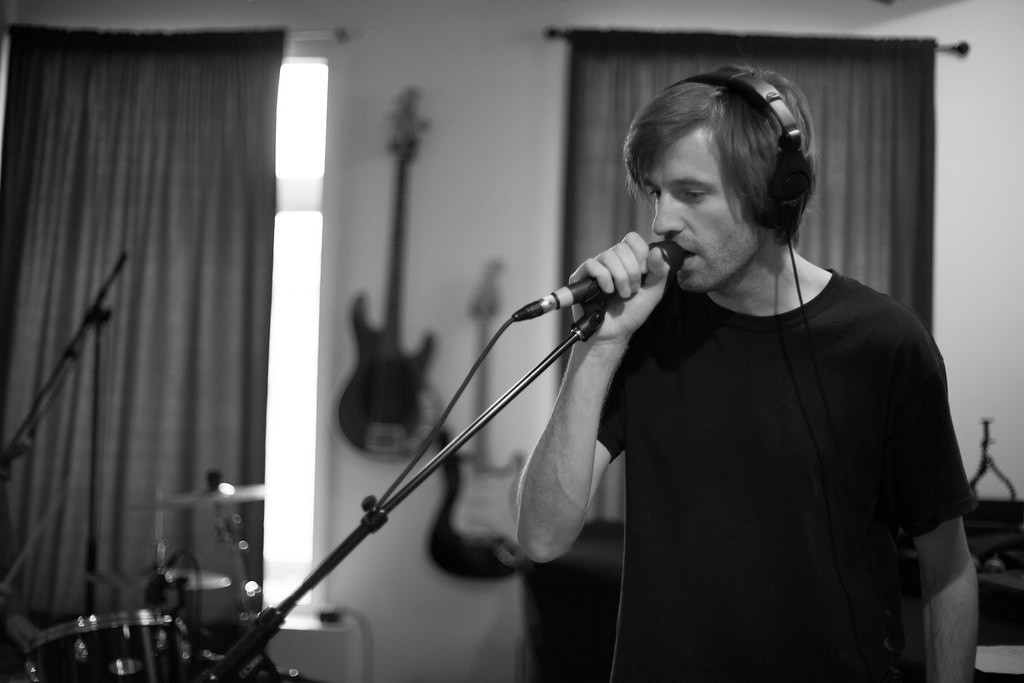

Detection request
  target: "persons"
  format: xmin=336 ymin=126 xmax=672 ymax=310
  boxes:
xmin=518 ymin=65 xmax=980 ymax=683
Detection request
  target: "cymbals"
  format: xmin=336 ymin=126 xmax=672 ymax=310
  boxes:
xmin=168 ymin=485 xmax=270 ymax=507
xmin=132 ymin=570 xmax=233 ymax=592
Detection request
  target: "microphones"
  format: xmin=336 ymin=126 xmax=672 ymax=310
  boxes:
xmin=512 ymin=240 xmax=685 ymax=323
xmin=146 ymin=557 xmax=178 ymax=607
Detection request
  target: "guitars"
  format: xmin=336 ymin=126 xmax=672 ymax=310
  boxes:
xmin=338 ymin=84 xmax=441 ymax=457
xmin=424 ymin=255 xmax=529 ymax=584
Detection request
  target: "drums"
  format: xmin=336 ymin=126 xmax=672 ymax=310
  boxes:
xmin=31 ymin=607 xmax=189 ymax=683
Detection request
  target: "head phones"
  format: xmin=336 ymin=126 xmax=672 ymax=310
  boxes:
xmin=672 ymin=69 xmax=813 ymax=233
